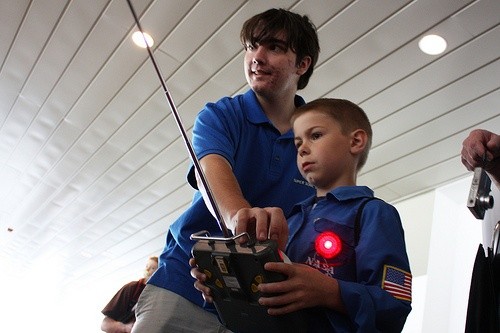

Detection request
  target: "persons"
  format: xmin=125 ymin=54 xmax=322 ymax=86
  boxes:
xmin=101 ymin=7 xmax=412 ymax=332
xmin=461 ymin=129 xmax=500 ymax=185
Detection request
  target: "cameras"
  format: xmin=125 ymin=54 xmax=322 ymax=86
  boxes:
xmin=467 ymin=167 xmax=494 ymax=220
